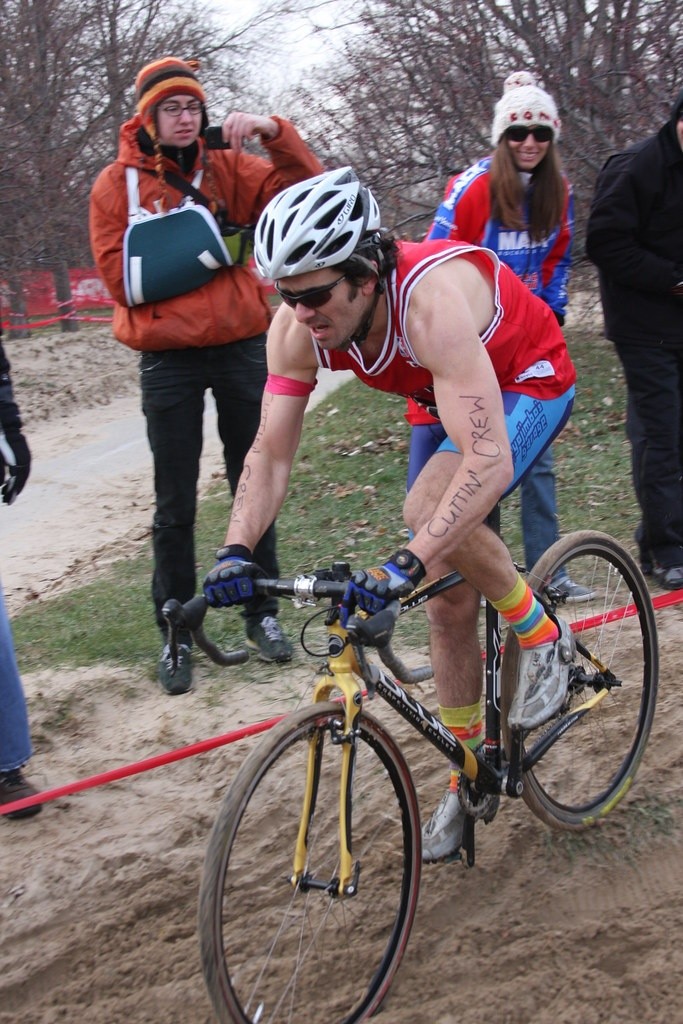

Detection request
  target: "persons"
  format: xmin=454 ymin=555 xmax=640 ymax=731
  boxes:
xmin=0 ymin=328 xmax=42 ymax=821
xmin=423 ymin=70 xmax=597 ymax=608
xmin=202 ymin=165 xmax=577 ymax=865
xmin=89 ymin=56 xmax=323 ymax=694
xmin=584 ymin=92 xmax=683 ymax=590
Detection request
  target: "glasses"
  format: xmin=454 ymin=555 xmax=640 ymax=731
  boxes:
xmin=504 ymin=125 xmax=553 ymax=143
xmin=274 ymin=275 xmax=346 ymax=309
xmin=162 ymin=103 xmax=203 ymax=116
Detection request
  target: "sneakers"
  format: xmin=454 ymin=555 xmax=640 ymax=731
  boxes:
xmin=558 ymin=578 xmax=596 ymax=603
xmin=158 ymin=632 xmax=193 ymax=694
xmin=507 ymin=614 xmax=576 ymax=731
xmin=421 ymin=788 xmax=466 ymax=865
xmin=640 ymin=564 xmax=683 ymax=590
xmin=0 ymin=768 xmax=43 ymax=820
xmin=245 ymin=616 xmax=293 ymax=662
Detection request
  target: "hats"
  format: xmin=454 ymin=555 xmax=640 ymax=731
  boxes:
xmin=490 ymin=71 xmax=561 ymax=148
xmin=135 ymin=57 xmax=217 ymax=214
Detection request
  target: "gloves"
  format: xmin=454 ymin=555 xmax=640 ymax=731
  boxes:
xmin=339 ymin=549 xmax=427 ymax=629
xmin=0 ymin=403 xmax=31 ymax=505
xmin=203 ymin=544 xmax=269 ymax=609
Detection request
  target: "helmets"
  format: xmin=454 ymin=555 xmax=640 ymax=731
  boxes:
xmin=253 ymin=166 xmax=381 ymax=280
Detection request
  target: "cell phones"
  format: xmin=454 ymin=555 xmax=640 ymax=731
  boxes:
xmin=205 ymin=126 xmax=245 ymax=150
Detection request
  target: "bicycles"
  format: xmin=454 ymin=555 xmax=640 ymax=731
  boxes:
xmin=159 ymin=528 xmax=662 ymax=1023
